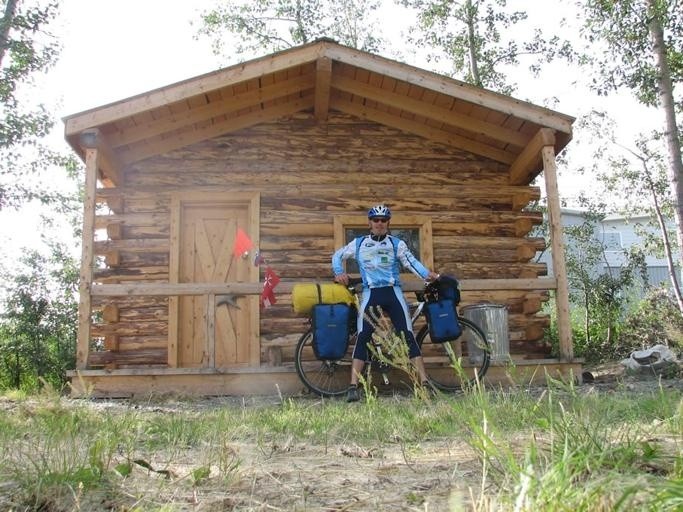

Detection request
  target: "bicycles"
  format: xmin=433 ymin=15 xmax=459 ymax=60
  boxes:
xmin=295 ymin=273 xmax=490 ymax=397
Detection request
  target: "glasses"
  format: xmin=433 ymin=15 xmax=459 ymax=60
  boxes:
xmin=370 ymin=217 xmax=389 ymax=223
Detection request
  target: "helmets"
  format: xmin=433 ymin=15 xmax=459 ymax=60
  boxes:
xmin=368 ymin=205 xmax=391 ymax=220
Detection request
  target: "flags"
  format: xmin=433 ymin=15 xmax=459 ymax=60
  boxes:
xmin=262 ymin=267 xmax=281 ymax=309
xmin=234 ymin=227 xmax=254 ymax=261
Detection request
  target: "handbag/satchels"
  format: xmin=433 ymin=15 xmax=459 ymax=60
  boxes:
xmin=423 ymin=275 xmax=462 ymax=343
xmin=292 ymin=283 xmax=355 ymax=314
xmin=312 ymin=302 xmax=353 ymax=360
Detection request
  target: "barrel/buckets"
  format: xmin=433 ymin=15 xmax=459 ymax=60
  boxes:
xmin=459 ymin=303 xmax=511 ymax=369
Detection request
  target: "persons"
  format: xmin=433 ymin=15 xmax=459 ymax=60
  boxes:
xmin=332 ymin=205 xmax=440 ymax=402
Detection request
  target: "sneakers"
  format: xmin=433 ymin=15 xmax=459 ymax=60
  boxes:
xmin=347 ymin=385 xmax=361 ymax=402
